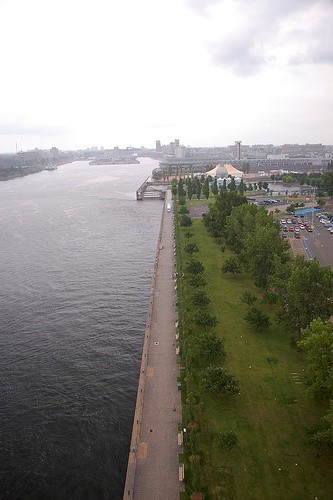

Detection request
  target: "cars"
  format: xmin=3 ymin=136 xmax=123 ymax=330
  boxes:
xmin=247 ymin=194 xmax=333 ymax=240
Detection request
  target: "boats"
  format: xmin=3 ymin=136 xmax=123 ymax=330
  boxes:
xmin=46 ymin=164 xmax=57 ymax=170
